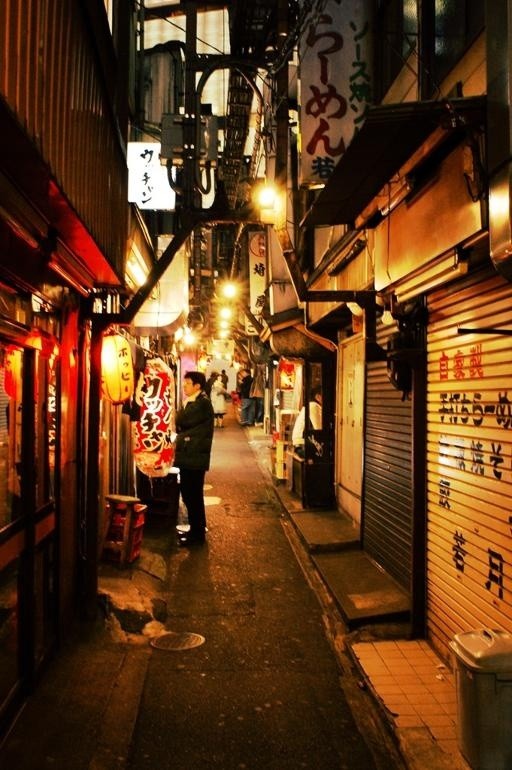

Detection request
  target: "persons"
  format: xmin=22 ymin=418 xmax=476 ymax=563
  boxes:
xmin=292 ymin=384 xmax=322 ymax=459
xmin=173 ymin=371 xmax=215 ymax=546
xmin=208 ymin=369 xmax=263 ymax=428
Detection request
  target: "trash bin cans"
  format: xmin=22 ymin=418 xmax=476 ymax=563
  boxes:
xmin=448 ymin=628 xmax=512 ymax=770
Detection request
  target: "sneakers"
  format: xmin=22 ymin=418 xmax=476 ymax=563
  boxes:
xmin=177 ymin=530 xmax=207 ymax=546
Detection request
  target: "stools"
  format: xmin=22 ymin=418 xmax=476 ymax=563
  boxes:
xmin=98 ymin=495 xmax=141 ymax=570
